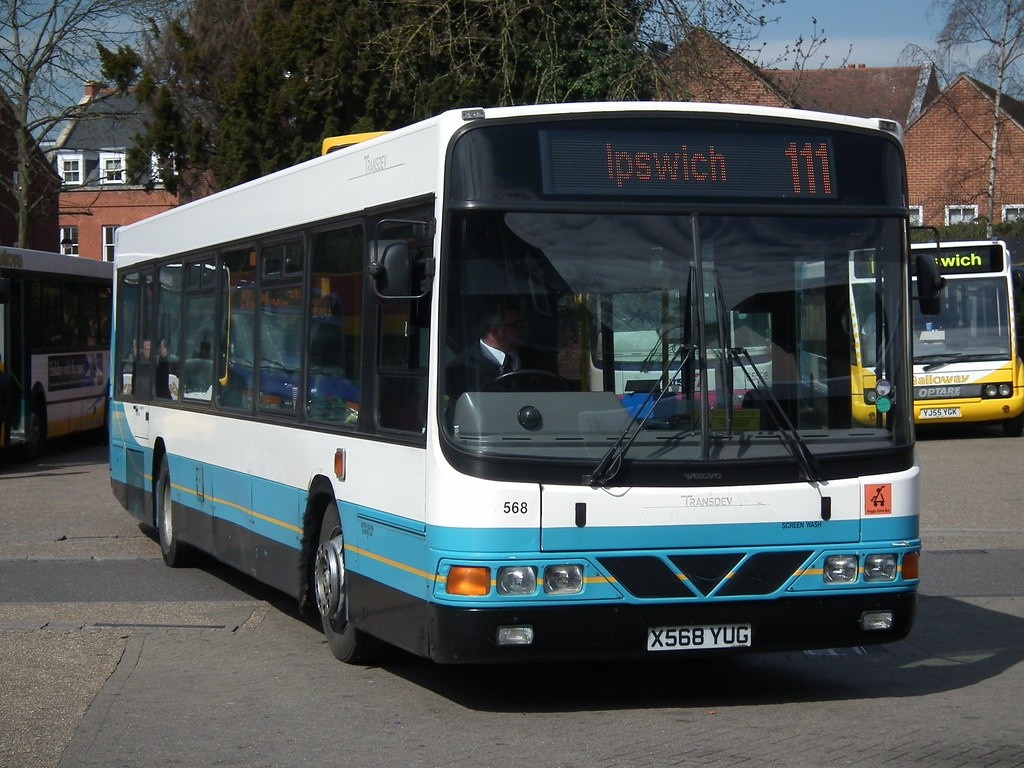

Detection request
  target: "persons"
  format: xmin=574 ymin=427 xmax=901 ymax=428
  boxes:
xmin=443 ymin=299 xmax=551 ymax=425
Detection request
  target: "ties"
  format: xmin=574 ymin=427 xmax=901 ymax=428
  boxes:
xmin=502 ymin=355 xmax=515 ymax=389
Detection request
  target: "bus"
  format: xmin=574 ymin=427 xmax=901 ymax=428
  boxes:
xmin=583 ymin=252 xmax=780 ymax=419
xmin=0 ymin=244 xmax=127 ymax=464
xmin=800 ymin=237 xmax=1024 ymax=438
xmin=107 ymin=99 xmax=948 ymax=668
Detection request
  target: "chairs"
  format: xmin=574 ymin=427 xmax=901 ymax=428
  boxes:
xmin=120 ymin=337 xmax=369 ymax=421
